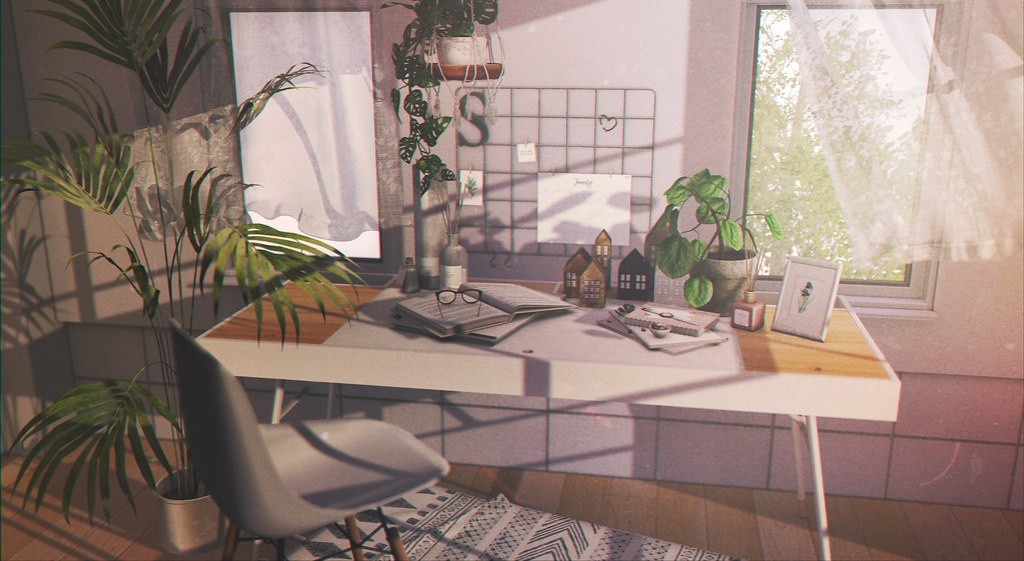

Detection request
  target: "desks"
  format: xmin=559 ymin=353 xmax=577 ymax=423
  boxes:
xmin=194 ymin=271 xmax=903 ymax=561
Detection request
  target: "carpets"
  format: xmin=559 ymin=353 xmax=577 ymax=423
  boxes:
xmin=286 ymin=485 xmax=748 ymax=561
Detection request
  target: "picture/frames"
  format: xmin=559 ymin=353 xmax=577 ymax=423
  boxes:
xmin=771 ymin=255 xmax=842 ymax=343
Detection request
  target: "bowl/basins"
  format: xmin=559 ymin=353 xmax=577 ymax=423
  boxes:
xmin=649 ymin=324 xmax=672 ymax=337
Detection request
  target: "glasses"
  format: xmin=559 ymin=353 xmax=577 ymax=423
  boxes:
xmin=434 ymin=289 xmax=483 ymax=318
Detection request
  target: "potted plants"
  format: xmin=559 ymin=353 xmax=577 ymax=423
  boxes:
xmin=1 ymin=0 xmax=372 ymax=561
xmin=380 ymin=0 xmax=500 ymax=199
xmin=645 ymin=169 xmax=785 ymax=317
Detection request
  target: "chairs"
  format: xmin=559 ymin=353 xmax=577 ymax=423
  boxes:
xmin=166 ymin=316 xmax=452 ymax=561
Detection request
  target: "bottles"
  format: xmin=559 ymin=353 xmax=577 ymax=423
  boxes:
xmin=730 ymin=291 xmax=766 ymax=332
xmin=401 ymin=256 xmax=419 ymax=294
